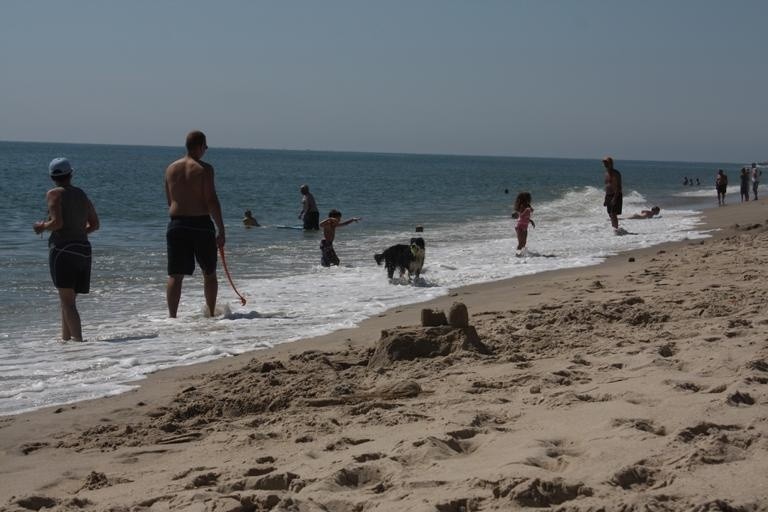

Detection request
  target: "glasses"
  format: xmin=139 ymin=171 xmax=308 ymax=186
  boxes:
xmin=204 ymin=145 xmax=209 ymax=150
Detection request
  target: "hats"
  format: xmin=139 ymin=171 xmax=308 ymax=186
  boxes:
xmin=48 ymin=157 xmax=74 ymax=177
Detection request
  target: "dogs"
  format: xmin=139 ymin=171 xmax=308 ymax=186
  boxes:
xmin=374 ymin=237 xmax=426 ymax=283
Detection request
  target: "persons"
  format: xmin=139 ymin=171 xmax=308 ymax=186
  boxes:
xmin=632 ymin=205 xmax=661 ymax=220
xmin=680 ymin=160 xmax=762 ymax=205
xmin=510 ymin=191 xmax=535 ymax=252
xmin=242 ymin=208 xmax=260 ymax=230
xmin=316 ymin=207 xmax=363 ymax=269
xmin=599 ymin=155 xmax=623 ymax=230
xmin=31 ymin=156 xmax=101 ymax=344
xmin=296 ymin=182 xmax=321 ymax=233
xmin=162 ymin=128 xmax=226 ymax=318
